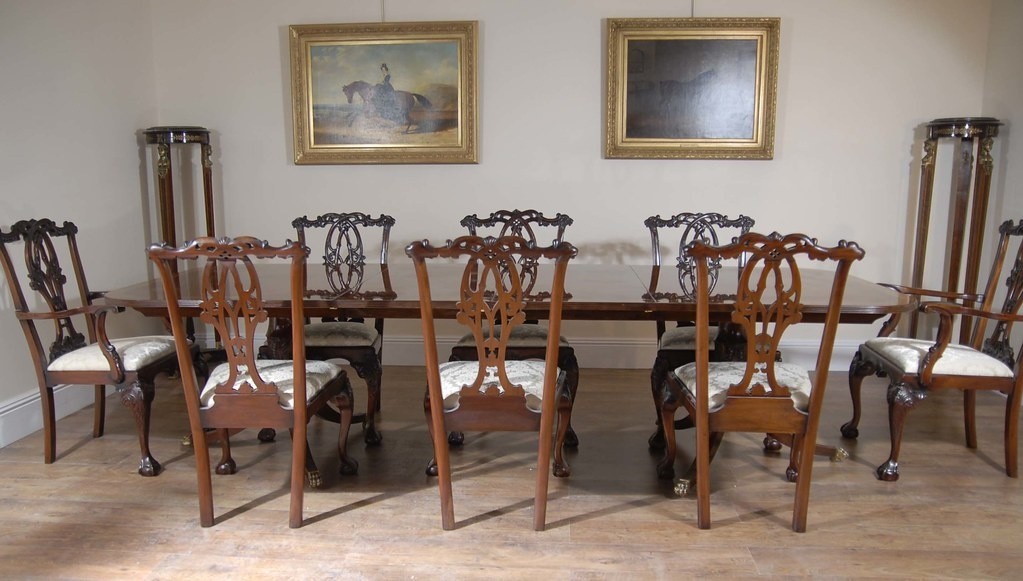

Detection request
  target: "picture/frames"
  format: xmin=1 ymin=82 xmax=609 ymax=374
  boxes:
xmin=604 ymin=16 xmax=773 ymax=161
xmin=287 ymin=22 xmax=477 ymax=165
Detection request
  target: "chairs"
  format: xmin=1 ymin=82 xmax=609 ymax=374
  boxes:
xmin=657 ymin=231 xmax=866 ymax=530
xmin=259 ymin=213 xmax=396 ymax=445
xmin=841 ymin=220 xmax=1022 ymax=483
xmin=409 ymin=235 xmax=579 ymax=532
xmin=0 ymin=217 xmax=209 ymax=478
xmin=424 ymin=208 xmax=580 ymax=447
xmin=642 ymin=212 xmax=783 ymax=477
xmin=145 ymin=232 xmax=358 ymax=529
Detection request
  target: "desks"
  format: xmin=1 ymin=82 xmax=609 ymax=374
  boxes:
xmin=106 ymin=260 xmax=918 ymax=496
